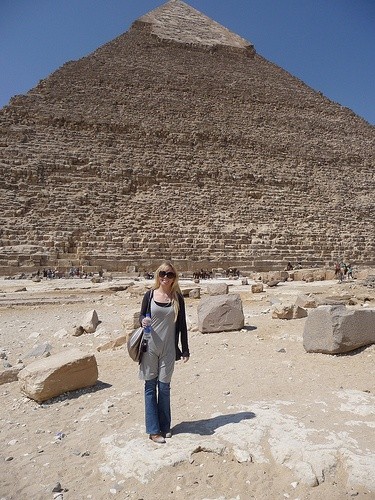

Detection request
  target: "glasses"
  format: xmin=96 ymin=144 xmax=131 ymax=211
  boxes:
xmin=159 ymin=271 xmax=176 ymax=279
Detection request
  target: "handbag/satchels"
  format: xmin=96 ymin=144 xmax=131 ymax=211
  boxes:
xmin=127 ymin=326 xmax=145 ymax=362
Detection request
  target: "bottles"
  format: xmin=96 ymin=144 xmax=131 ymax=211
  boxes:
xmin=143 ymin=314 xmax=151 ymax=340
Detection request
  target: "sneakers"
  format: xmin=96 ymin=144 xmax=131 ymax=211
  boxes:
xmin=149 ymin=432 xmax=171 ymax=442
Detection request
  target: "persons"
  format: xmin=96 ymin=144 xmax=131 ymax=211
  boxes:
xmin=285 ymin=261 xmax=303 ymax=271
xmin=37 ymin=268 xmax=62 ymax=279
xmin=143 ymin=270 xmax=155 ymax=279
xmin=138 ymin=260 xmax=190 ymax=443
xmin=334 ymin=261 xmax=354 ymax=283
xmin=193 ymin=267 xmax=240 ymax=279
xmin=66 ymin=266 xmax=81 ymax=278
